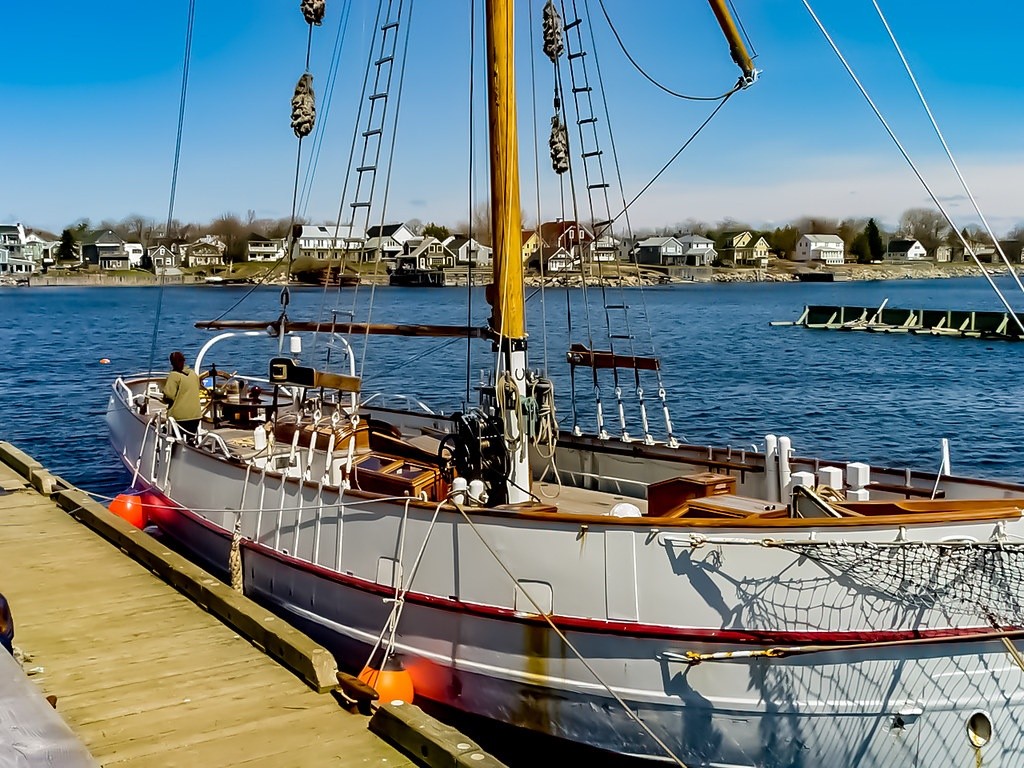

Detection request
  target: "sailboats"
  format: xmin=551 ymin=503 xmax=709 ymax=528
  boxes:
xmin=103 ymin=1 xmax=1023 ymax=768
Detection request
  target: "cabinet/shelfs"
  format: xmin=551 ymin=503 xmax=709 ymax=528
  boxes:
xmin=340 ymin=453 xmax=459 ymax=504
xmin=663 ymin=494 xmax=790 ymax=519
xmin=271 ymin=412 xmax=370 ymax=450
xmin=301 ymin=446 xmax=372 ymax=488
xmin=214 ymin=427 xmax=302 ymax=480
xmin=647 ymin=472 xmax=736 ymax=517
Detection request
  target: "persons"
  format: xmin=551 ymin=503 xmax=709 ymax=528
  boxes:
xmin=162 ymin=352 xmax=203 ymax=446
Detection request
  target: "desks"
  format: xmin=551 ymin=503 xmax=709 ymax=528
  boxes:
xmin=219 ymin=393 xmax=294 ymax=430
